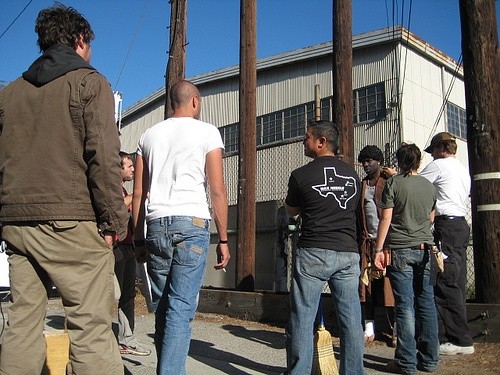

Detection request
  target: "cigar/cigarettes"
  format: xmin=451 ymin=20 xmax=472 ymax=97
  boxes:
xmin=223 ymin=268 xmax=226 ymax=272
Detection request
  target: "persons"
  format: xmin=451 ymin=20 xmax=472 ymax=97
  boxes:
xmin=114 ymin=272 xmax=151 ymax=356
xmin=0 ymin=0 xmax=131 ymax=375
xmin=373 ymin=142 xmax=438 ymax=375
xmin=383 ymin=131 xmax=474 ymax=355
xmin=113 ymin=151 xmax=136 ymax=333
xmin=131 ymin=80 xmax=231 ymax=375
xmin=357 ymin=145 xmax=388 ymax=347
xmin=284 ymin=120 xmax=368 ymax=375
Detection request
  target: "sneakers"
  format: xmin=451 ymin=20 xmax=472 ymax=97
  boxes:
xmin=118 ymin=342 xmax=151 ymax=356
xmin=438 ymin=341 xmax=474 ymax=356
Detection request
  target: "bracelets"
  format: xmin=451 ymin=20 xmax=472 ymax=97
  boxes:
xmin=219 ymin=240 xmax=228 ymax=244
xmin=376 ymin=248 xmax=383 ymax=252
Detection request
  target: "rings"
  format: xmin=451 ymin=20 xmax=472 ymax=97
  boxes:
xmin=386 ymin=169 xmax=388 ymax=172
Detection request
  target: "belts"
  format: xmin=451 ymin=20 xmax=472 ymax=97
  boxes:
xmin=438 ymin=214 xmax=466 ymax=221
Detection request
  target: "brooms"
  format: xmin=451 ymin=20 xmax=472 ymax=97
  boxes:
xmin=311 ymin=297 xmax=339 ymax=375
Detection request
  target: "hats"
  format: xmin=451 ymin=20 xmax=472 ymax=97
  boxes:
xmin=423 ymin=132 xmax=455 ymax=153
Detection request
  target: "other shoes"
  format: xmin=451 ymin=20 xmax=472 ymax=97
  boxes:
xmin=365 ymin=333 xmax=375 ymax=343
xmin=386 ymin=360 xmax=408 ymax=374
